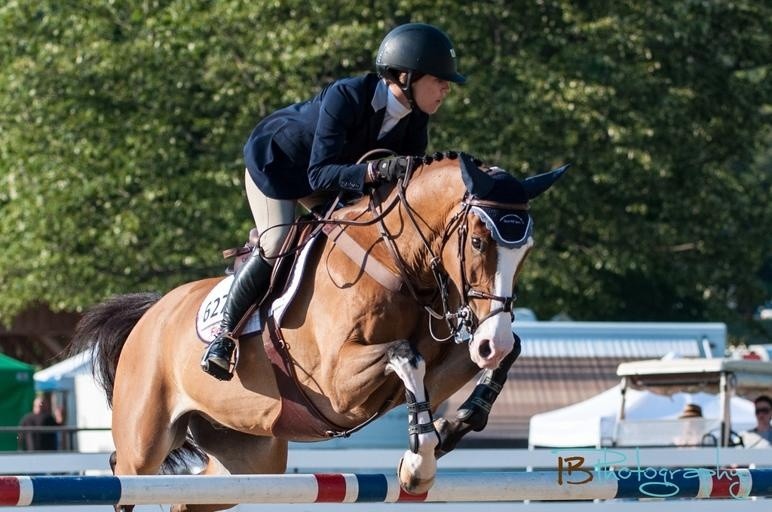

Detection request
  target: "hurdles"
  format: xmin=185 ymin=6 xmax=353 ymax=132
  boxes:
xmin=0 ymin=451 xmax=771 ymax=507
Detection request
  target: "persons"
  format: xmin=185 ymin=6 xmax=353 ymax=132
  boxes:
xmin=673 ymin=405 xmax=707 ymax=446
xmin=732 ymin=396 xmax=772 ymax=447
xmin=201 ymin=23 xmax=467 ymax=381
xmin=15 ymin=395 xmax=58 ymax=450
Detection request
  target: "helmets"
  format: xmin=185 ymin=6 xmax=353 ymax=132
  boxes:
xmin=375 ymin=21 xmax=466 ymax=84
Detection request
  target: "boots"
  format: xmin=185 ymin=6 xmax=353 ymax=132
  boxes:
xmin=200 ymin=245 xmax=275 ymax=383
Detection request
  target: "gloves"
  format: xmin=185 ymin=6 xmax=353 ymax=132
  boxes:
xmin=371 ymin=155 xmax=418 ymax=182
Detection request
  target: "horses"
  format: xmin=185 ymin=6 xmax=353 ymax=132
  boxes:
xmin=61 ymin=149 xmax=570 ymax=512
